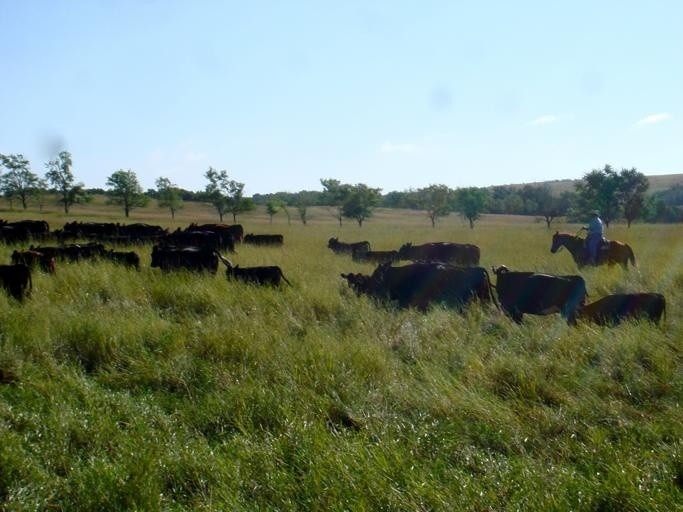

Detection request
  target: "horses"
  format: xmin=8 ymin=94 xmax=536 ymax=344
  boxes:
xmin=550 ymin=231 xmax=638 ymax=272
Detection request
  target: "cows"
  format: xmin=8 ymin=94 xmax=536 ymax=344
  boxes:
xmin=491 ymin=265 xmax=589 ymax=327
xmin=243 ymin=232 xmax=284 ymax=246
xmin=342 ymin=242 xmax=500 ymax=315
xmin=579 ymin=292 xmax=666 ymax=329
xmin=0 ymin=265 xmax=32 ymax=303
xmin=225 ymin=264 xmax=293 ymax=290
xmin=0 ymin=219 xmax=244 ymax=277
xmin=327 ymin=238 xmax=371 ymax=257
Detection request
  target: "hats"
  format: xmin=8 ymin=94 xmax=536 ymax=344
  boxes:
xmin=592 ymin=210 xmax=599 ymax=215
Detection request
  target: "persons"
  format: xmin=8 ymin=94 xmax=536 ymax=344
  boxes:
xmin=583 ymin=210 xmax=603 ymax=267
